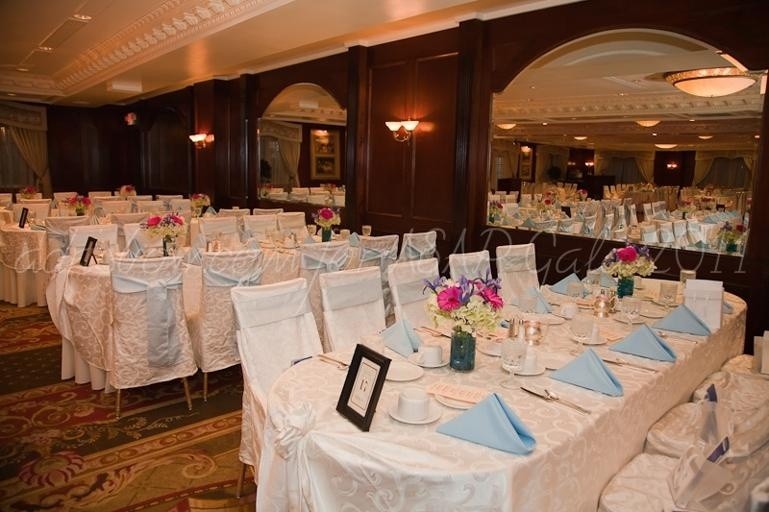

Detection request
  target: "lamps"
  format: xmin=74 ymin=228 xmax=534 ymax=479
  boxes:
xmin=662 ymin=65 xmax=759 ymax=98
xmin=385 ymin=120 xmax=421 ymax=146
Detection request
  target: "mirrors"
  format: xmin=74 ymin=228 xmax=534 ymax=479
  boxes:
xmin=483 ymin=30 xmax=769 ymax=260
xmin=255 ymin=82 xmax=349 ymax=209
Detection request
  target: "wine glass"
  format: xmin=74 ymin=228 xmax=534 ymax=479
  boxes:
xmin=306 ymin=223 xmax=373 ymax=242
xmin=473 ymin=269 xmax=700 ymax=390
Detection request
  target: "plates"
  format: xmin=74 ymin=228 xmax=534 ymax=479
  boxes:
xmin=389 ymin=403 xmax=442 ymax=424
xmin=407 ymin=351 xmax=451 ymax=368
xmin=435 ymin=384 xmax=487 ymax=410
xmin=384 ymin=361 xmax=424 ymax=381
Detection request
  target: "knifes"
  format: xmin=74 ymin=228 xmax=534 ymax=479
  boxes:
xmin=520 ymin=386 xmax=587 ymax=416
xmin=421 ymin=326 xmax=452 ymax=338
xmin=318 ymin=354 xmax=351 ymax=367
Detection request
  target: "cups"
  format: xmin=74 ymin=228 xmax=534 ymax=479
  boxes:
xmin=397 ymin=388 xmax=429 ymax=420
xmin=27 ymin=206 xmax=40 ymax=230
xmin=416 ymin=346 xmax=442 ymax=363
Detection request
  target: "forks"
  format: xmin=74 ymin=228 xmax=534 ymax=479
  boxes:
xmin=544 ymin=389 xmax=590 ymax=415
xmin=320 ymin=358 xmax=348 ymax=370
xmin=413 ymin=328 xmax=441 ymax=337
